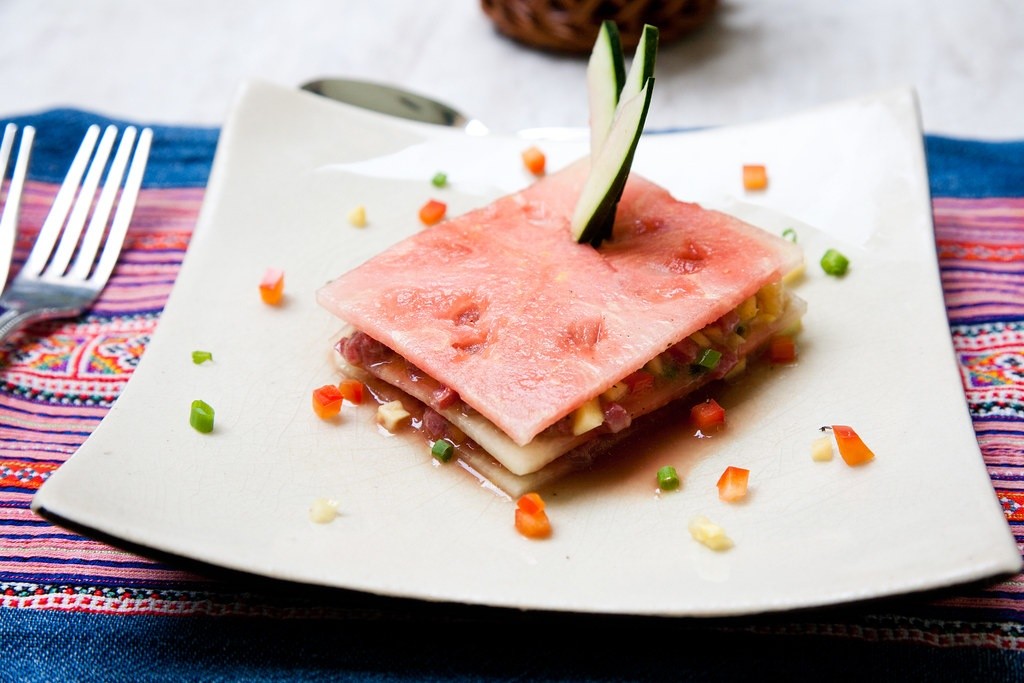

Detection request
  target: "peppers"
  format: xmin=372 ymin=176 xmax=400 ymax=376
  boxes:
xmin=187 ymin=145 xmax=873 ymax=537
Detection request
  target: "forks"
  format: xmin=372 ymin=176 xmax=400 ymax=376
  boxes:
xmin=0 ymin=123 xmax=154 ymax=341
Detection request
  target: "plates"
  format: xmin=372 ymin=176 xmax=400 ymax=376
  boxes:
xmin=30 ymin=74 xmax=1022 ymax=618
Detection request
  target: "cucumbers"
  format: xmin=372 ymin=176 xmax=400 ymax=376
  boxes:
xmin=569 ymin=18 xmax=659 ymax=248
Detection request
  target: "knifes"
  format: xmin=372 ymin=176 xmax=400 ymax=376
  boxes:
xmin=299 ymin=79 xmax=467 ymax=132
